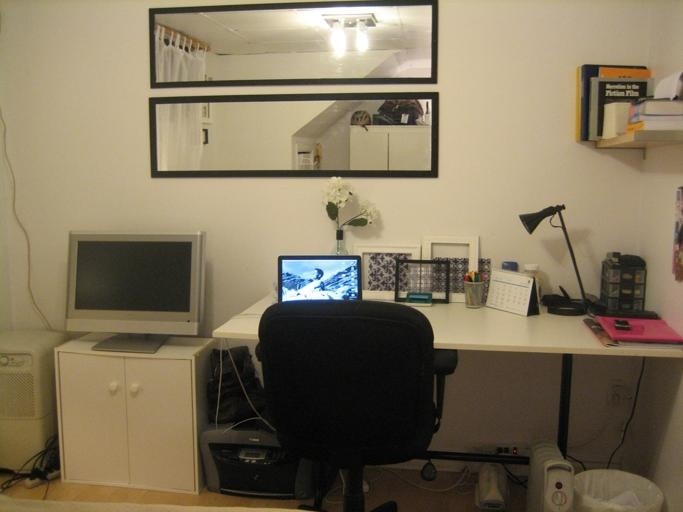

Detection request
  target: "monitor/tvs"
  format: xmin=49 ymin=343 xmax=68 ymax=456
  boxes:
xmin=59 ymin=229 xmax=205 ymax=353
xmin=277 ymin=255 xmax=361 ymax=302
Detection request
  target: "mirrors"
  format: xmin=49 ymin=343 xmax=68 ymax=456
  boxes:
xmin=147 ymin=0 xmax=439 ymax=88
xmin=148 ymin=91 xmax=441 ymax=178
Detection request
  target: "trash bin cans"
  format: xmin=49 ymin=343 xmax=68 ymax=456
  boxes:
xmin=574 ymin=469 xmax=664 ymax=512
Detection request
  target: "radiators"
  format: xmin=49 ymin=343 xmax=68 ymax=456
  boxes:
xmin=525 ymin=437 xmax=575 ymax=512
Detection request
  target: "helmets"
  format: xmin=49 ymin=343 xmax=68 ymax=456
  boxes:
xmin=351 ymin=109 xmax=371 ymax=125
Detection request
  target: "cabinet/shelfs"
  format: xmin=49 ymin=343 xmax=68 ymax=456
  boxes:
xmin=54 ymin=332 xmax=214 ymax=496
xmin=348 ymin=123 xmax=431 ymax=171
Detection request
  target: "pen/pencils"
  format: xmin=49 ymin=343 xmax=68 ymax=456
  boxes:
xmin=463 ymin=271 xmax=484 ymax=306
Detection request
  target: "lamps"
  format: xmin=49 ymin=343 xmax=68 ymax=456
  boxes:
xmin=518 ymin=203 xmax=589 ymax=316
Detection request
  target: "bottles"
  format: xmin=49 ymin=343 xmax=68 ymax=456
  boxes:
xmin=522 ymin=263 xmax=541 ymax=304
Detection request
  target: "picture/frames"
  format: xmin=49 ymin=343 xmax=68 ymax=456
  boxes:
xmin=421 ymin=234 xmax=480 ymax=302
xmin=352 ymin=244 xmax=421 ymax=299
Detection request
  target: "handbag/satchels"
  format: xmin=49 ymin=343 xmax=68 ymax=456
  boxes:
xmin=205 ymin=344 xmax=267 ymax=431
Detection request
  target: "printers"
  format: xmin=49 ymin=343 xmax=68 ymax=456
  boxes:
xmin=201 ymin=422 xmax=306 ymax=500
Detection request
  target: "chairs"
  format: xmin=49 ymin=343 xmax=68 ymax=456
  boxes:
xmin=254 ymin=298 xmax=459 ymax=512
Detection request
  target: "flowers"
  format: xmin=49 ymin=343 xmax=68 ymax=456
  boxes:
xmin=323 ymin=176 xmax=378 ymax=230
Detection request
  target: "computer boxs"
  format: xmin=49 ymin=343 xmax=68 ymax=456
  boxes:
xmin=0 ymin=330 xmax=65 ymax=475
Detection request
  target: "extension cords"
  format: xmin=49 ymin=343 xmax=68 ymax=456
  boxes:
xmin=25 ymin=470 xmax=58 ymax=490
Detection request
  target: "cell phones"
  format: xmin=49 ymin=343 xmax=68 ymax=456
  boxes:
xmin=615 ymin=319 xmax=630 ymax=329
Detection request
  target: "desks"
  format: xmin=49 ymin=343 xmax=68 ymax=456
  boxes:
xmin=209 ymin=289 xmax=682 ymax=465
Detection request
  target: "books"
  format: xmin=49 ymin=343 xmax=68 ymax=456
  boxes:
xmin=572 ymin=63 xmax=683 ymax=143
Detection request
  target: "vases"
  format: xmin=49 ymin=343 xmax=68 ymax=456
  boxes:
xmin=332 ymin=228 xmax=348 ymax=256
